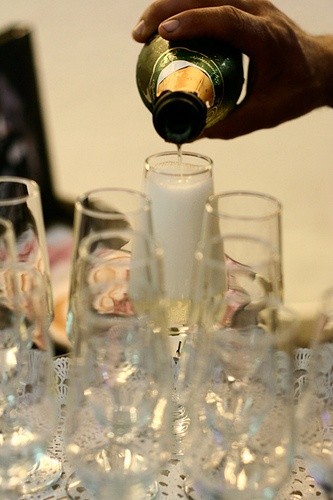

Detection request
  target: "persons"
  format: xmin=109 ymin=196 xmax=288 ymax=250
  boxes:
xmin=131 ymin=0 xmax=333 ymax=139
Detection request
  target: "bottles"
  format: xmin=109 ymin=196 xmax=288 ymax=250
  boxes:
xmin=137 ymin=32 xmax=245 ymax=144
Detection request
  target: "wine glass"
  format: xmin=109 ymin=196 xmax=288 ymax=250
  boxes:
xmin=0 ymin=151 xmax=333 ymax=500
